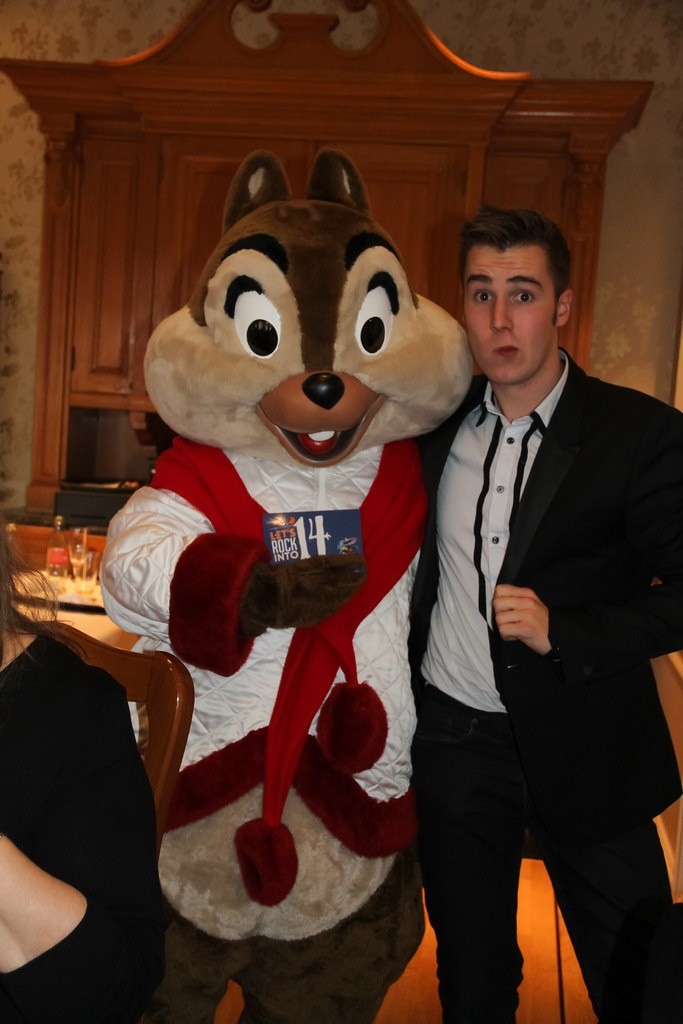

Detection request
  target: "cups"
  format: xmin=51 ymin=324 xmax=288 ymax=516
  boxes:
xmin=80 ymin=548 xmax=99 ymax=595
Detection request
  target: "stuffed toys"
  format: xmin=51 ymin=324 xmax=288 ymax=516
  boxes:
xmin=100 ymin=147 xmax=473 ymax=1024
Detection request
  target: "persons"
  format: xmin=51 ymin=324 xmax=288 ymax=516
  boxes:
xmin=408 ymin=204 xmax=683 ymax=1024
xmin=0 ymin=522 xmax=167 ymax=1024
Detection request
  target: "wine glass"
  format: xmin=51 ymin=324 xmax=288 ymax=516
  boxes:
xmin=66 ymin=526 xmax=87 ymax=583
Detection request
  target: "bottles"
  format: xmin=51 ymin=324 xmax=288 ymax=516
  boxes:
xmin=46 ymin=516 xmax=70 ymax=578
